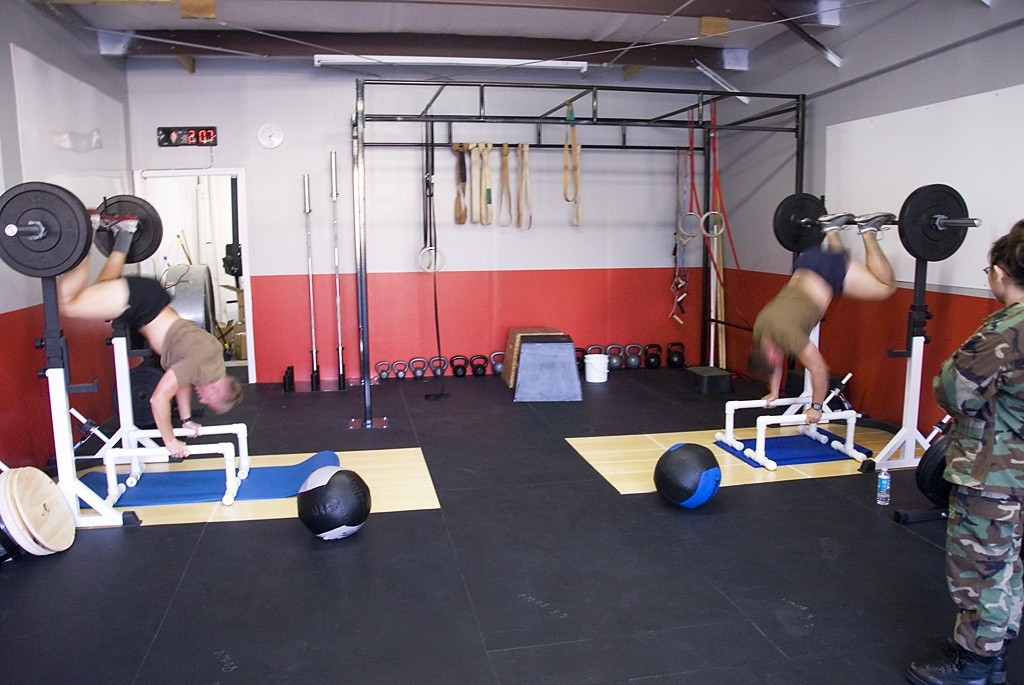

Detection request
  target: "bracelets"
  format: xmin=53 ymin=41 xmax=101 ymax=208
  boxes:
xmin=181 ymin=416 xmax=192 ymax=424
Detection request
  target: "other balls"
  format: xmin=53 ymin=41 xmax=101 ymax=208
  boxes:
xmin=653 ymin=441 xmax=723 ymax=511
xmin=292 ymin=466 xmax=372 ymax=542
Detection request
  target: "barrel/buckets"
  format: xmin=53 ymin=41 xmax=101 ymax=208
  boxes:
xmin=584 ymin=354 xmax=609 ymax=383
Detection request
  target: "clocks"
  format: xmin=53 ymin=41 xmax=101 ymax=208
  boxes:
xmin=258 ymin=122 xmax=284 ymax=149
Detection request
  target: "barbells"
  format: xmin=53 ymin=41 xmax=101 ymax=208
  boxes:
xmin=771 ymin=182 xmax=982 ymax=263
xmin=0 ymin=180 xmax=165 ymax=279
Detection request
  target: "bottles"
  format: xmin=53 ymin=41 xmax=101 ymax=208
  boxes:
xmin=877 ymin=468 xmax=891 ymax=506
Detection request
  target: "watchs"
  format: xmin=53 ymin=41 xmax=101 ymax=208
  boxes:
xmin=810 ymin=403 xmax=824 ymax=409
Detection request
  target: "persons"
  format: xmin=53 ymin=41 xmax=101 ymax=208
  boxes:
xmin=748 ymin=213 xmax=899 ymax=425
xmin=906 ymin=218 xmax=1023 ymax=685
xmin=55 ymin=212 xmax=243 ymax=458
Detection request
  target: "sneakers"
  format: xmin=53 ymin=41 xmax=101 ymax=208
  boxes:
xmin=854 ymin=212 xmax=896 ymax=235
xmin=818 ymin=212 xmax=855 ymax=233
xmin=89 ymin=215 xmax=101 ymax=230
xmin=104 ymin=218 xmax=138 ymax=237
xmin=905 ymin=651 xmax=1002 ymax=685
xmin=944 ymin=636 xmax=1008 ymax=684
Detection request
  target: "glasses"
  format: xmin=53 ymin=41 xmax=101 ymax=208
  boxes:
xmin=984 ymin=265 xmax=1012 ymax=279
